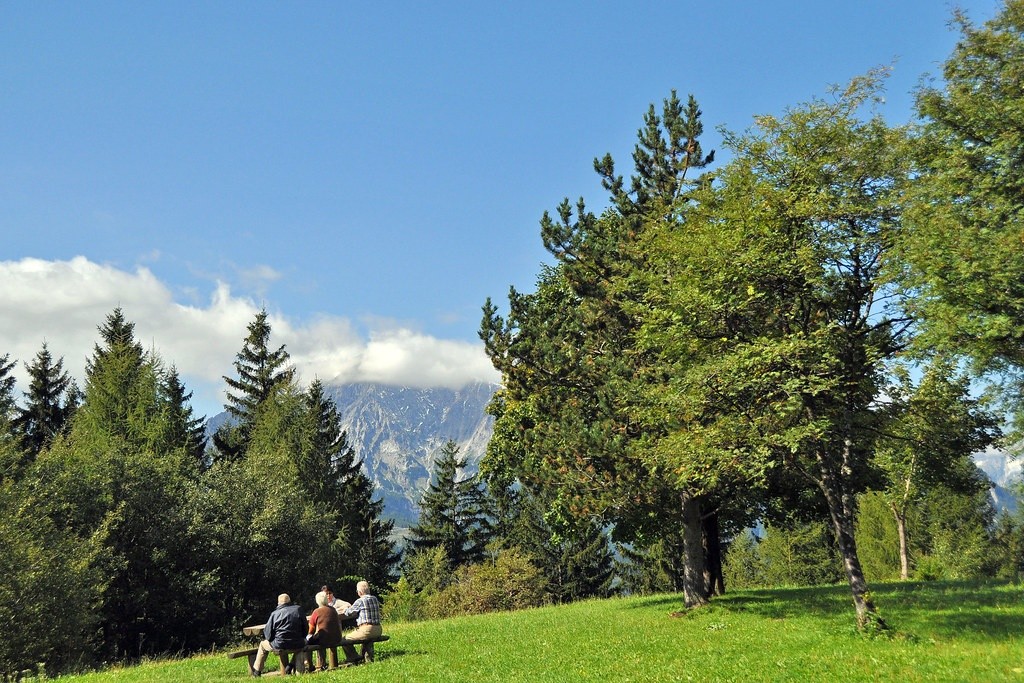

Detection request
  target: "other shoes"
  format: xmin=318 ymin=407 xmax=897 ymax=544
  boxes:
xmin=305 ymin=665 xmax=316 ymax=674
xmin=253 ymin=672 xmax=259 ymax=677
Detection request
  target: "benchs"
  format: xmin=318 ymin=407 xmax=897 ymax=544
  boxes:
xmin=273 ymin=636 xmax=390 ymax=678
xmin=228 ymin=647 xmax=258 ymax=675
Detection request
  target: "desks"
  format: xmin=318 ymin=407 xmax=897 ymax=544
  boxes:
xmin=243 ymin=612 xmax=352 ymax=676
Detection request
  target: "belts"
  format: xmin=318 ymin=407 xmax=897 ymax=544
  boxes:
xmin=362 ymin=623 xmax=380 ymax=625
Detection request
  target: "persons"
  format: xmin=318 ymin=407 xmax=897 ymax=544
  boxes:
xmin=342 ymin=581 xmax=384 ymax=665
xmin=305 ymin=591 xmax=341 ymax=672
xmin=319 ymin=584 xmax=351 ymax=614
xmin=252 ymin=593 xmax=310 ymax=678
